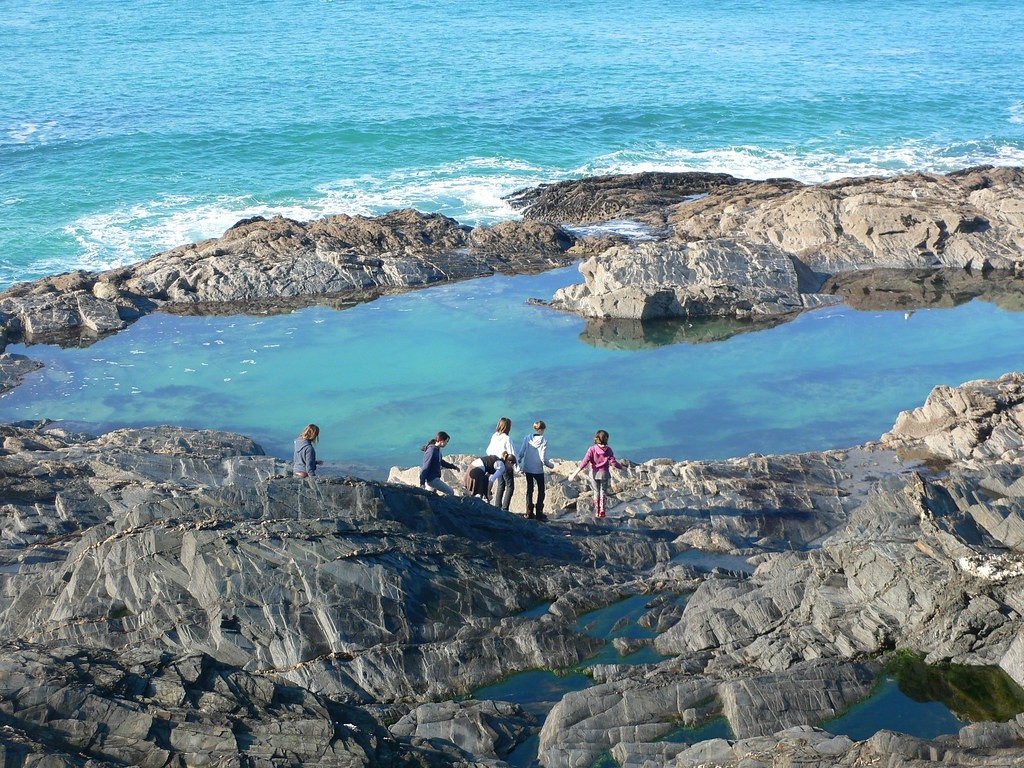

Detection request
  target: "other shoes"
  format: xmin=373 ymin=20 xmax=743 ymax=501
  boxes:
xmin=599 ymin=510 xmax=606 ymax=517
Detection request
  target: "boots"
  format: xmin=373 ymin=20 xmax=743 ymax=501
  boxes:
xmin=526 ymin=504 xmax=534 ymax=518
xmin=536 ymin=503 xmax=547 ymax=520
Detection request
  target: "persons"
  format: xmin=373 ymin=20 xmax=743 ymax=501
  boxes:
xmin=568 ymin=430 xmax=627 ymax=518
xmin=293 ymin=424 xmax=324 ymax=476
xmin=420 ymin=431 xmax=460 ymax=495
xmin=464 ymin=418 xmax=518 ymax=512
xmin=519 ymin=421 xmax=554 ymax=522
